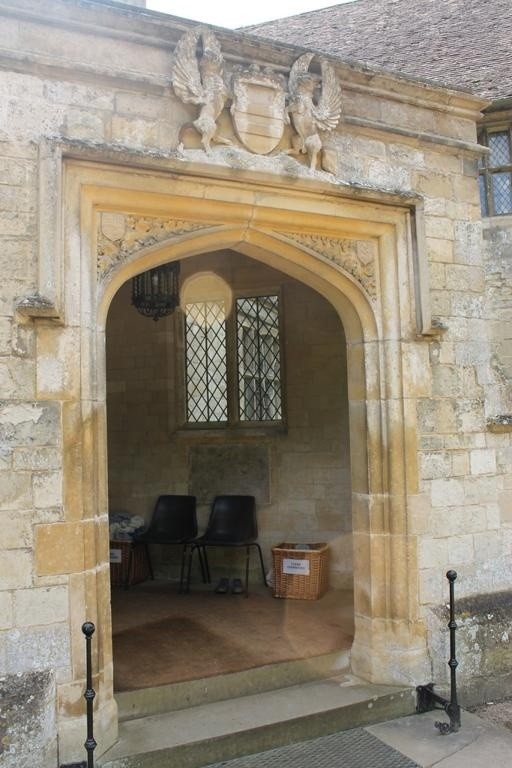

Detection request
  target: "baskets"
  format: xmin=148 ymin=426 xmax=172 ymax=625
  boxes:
xmin=110 ymin=535 xmax=150 ymax=587
xmin=270 ymin=538 xmax=329 ymax=602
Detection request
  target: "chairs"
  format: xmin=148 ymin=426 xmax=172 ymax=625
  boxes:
xmin=133 ymin=495 xmax=269 ymax=597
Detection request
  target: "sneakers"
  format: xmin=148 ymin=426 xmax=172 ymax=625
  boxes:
xmin=212 ymin=578 xmax=245 ymax=595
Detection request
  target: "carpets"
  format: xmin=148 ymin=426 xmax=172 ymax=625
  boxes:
xmin=200 ymin=726 xmax=422 ymax=767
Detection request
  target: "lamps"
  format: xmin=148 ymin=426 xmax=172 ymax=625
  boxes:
xmin=133 ymin=258 xmax=181 ymax=323
